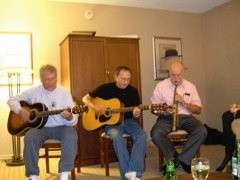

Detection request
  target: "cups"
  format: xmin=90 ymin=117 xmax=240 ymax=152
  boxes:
xmin=190 ymin=158 xmax=210 ymax=180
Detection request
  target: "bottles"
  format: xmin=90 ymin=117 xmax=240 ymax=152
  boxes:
xmin=231 ymin=138 xmax=240 ymax=180
xmin=163 ymin=160 xmax=179 ymax=180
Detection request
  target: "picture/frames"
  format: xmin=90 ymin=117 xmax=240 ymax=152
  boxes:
xmin=0 ymin=31 xmax=34 ymax=86
xmin=152 ymin=35 xmax=182 ymax=81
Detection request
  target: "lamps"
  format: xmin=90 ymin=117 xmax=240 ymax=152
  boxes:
xmin=0 ymin=52 xmax=34 ymax=167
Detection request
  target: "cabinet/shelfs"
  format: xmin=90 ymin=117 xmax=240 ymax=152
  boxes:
xmin=59 ymin=33 xmax=143 ymax=167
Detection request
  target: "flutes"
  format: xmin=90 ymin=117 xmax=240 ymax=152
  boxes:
xmin=172 ymin=81 xmax=180 ymax=132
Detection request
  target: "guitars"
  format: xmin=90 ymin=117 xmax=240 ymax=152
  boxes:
xmin=7 ymin=101 xmax=91 ymax=137
xmin=82 ymin=95 xmax=170 ymax=132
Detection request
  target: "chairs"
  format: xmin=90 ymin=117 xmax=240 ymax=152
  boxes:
xmin=98 ymin=126 xmax=146 ymax=175
xmin=158 ymin=129 xmax=200 ymax=171
xmin=40 ymin=139 xmax=81 ymax=180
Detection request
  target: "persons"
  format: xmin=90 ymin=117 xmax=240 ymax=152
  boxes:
xmin=216 ymin=103 xmax=240 ymax=173
xmin=7 ymin=64 xmax=79 ymax=180
xmin=150 ymin=61 xmax=206 ymax=174
xmin=82 ymin=67 xmax=147 ymax=180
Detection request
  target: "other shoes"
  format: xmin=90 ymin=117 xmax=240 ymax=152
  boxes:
xmin=181 ymin=161 xmax=191 ymax=174
xmin=162 ymin=160 xmax=179 ymax=176
